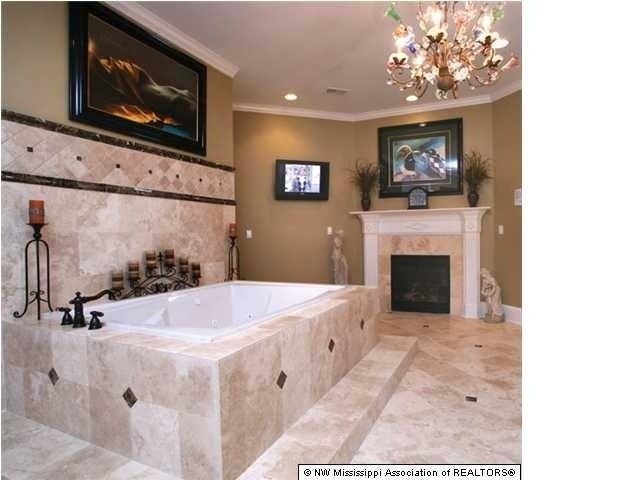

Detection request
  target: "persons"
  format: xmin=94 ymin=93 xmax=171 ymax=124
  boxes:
xmin=292 ymin=167 xmax=312 ymax=192
xmin=332 ymin=229 xmax=348 ymax=284
xmin=480 ymin=268 xmax=503 ymax=320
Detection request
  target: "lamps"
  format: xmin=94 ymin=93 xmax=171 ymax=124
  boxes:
xmin=382 ymin=2 xmax=519 ymax=105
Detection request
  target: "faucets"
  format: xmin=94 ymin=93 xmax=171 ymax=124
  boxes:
xmin=65 ymin=288 xmax=113 ymax=329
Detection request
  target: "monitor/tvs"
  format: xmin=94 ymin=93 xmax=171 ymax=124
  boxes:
xmin=275 ymin=159 xmax=329 ymax=201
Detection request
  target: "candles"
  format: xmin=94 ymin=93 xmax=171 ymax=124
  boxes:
xmin=110 ymin=247 xmax=201 ymax=288
xmin=28 ymin=199 xmax=46 ymax=223
xmin=229 ymin=223 xmax=238 ymax=236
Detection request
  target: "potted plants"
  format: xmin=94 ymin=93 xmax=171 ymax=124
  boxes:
xmin=346 ymin=159 xmax=380 ymax=211
xmin=460 ymin=149 xmax=495 ymax=206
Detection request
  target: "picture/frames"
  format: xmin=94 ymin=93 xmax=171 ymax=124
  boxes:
xmin=68 ymin=1 xmax=209 ymax=157
xmin=375 ymin=116 xmax=465 ymax=198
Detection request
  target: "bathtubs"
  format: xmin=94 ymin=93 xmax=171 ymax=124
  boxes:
xmin=36 ymin=279 xmax=348 ymax=344
